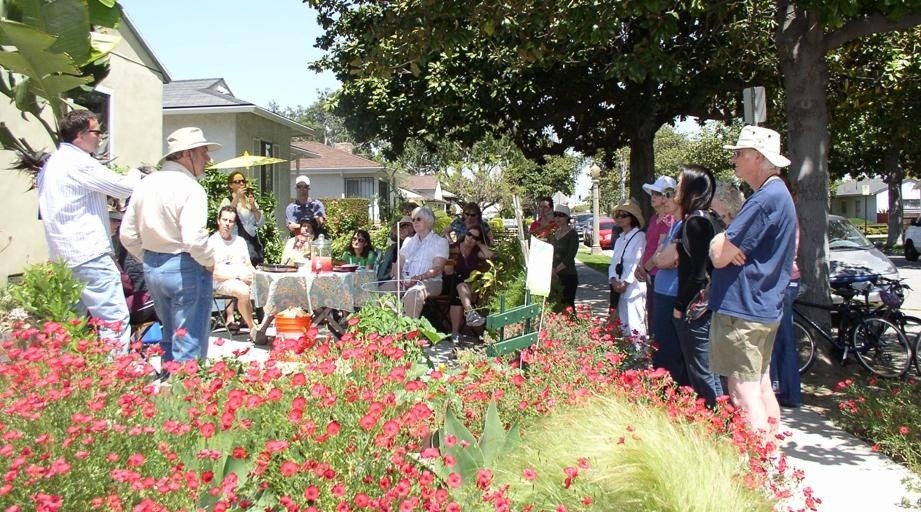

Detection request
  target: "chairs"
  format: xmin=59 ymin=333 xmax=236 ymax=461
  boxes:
xmin=211 ymin=292 xmax=236 ymax=341
xmin=130 ymin=321 xmax=160 ymax=377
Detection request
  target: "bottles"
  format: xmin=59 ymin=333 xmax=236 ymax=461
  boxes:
xmin=310 ymin=234 xmax=334 ymax=274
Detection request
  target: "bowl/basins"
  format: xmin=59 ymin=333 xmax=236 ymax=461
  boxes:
xmin=272 ymin=315 xmax=311 ymax=333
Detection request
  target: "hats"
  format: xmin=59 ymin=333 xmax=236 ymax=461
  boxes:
xmin=612 ymin=199 xmax=645 ymax=228
xmin=553 ymin=205 xmax=570 ymax=218
xmin=642 ymin=176 xmax=676 ymax=195
xmin=158 ymin=127 xmax=222 ymax=165
xmin=296 ymin=175 xmax=310 ymax=186
xmin=723 ymin=125 xmax=791 ymax=167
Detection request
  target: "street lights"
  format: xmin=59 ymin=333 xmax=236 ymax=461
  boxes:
xmin=591 ymin=163 xmax=605 ymax=254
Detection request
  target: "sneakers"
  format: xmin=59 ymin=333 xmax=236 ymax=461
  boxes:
xmin=466 ymin=314 xmax=485 ymax=327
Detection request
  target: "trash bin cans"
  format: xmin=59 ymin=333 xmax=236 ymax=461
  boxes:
xmin=274 ymin=313 xmax=313 ymax=341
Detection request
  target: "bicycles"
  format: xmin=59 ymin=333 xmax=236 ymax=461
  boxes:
xmin=790 ymin=274 xmax=921 ymax=379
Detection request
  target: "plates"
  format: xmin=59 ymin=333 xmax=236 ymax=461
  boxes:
xmin=335 ymin=265 xmax=359 ymax=272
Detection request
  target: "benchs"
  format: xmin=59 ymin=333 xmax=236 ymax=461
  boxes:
xmin=427 ymin=289 xmax=480 ymax=324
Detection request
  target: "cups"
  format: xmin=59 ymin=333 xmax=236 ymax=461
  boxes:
xmin=247 ymin=187 xmax=255 ymax=196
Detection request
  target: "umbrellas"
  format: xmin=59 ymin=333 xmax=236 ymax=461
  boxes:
xmin=206 ymin=151 xmax=288 ymax=177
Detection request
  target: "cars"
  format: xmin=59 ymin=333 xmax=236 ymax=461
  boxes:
xmin=573 ymin=213 xmax=616 ymax=249
xmin=828 ymin=213 xmax=905 ymax=318
xmin=903 ymin=213 xmax=921 ymax=262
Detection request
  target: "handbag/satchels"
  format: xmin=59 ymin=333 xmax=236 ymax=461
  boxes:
xmin=609 ymin=264 xmax=622 ymax=315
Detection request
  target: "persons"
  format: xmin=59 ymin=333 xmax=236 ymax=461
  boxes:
xmin=609 ymin=164 xmax=726 ymax=403
xmin=39 ymin=110 xmax=135 ymax=362
xmin=207 ymin=207 xmax=256 ymax=331
xmin=118 ymin=124 xmax=218 ymax=381
xmin=546 ymin=203 xmax=580 ymax=316
xmin=768 ymin=223 xmax=803 ymax=409
xmin=709 ymin=125 xmax=800 ymax=451
xmin=284 ymin=175 xmax=325 ymax=241
xmin=528 ymin=197 xmax=554 ymax=248
xmin=280 ymin=215 xmax=318 ymax=267
xmin=109 ymin=204 xmax=163 ymax=327
xmin=218 ymin=171 xmax=266 ymax=330
xmin=377 ymin=202 xmax=494 ymax=344
xmin=332 ymin=230 xmax=378 ymax=318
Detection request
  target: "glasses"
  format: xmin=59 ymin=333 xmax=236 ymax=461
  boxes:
xmin=466 ymin=231 xmax=479 ymax=241
xmin=615 ymin=214 xmax=630 ymax=218
xmin=663 ymin=191 xmax=674 ymax=198
xmin=553 ymin=213 xmax=565 ymax=217
xmin=413 ymin=218 xmax=421 ymax=222
xmin=230 ymin=180 xmax=245 ymax=183
xmin=352 ymin=238 xmax=364 ymax=242
xmin=465 ymin=212 xmax=477 ymax=216
